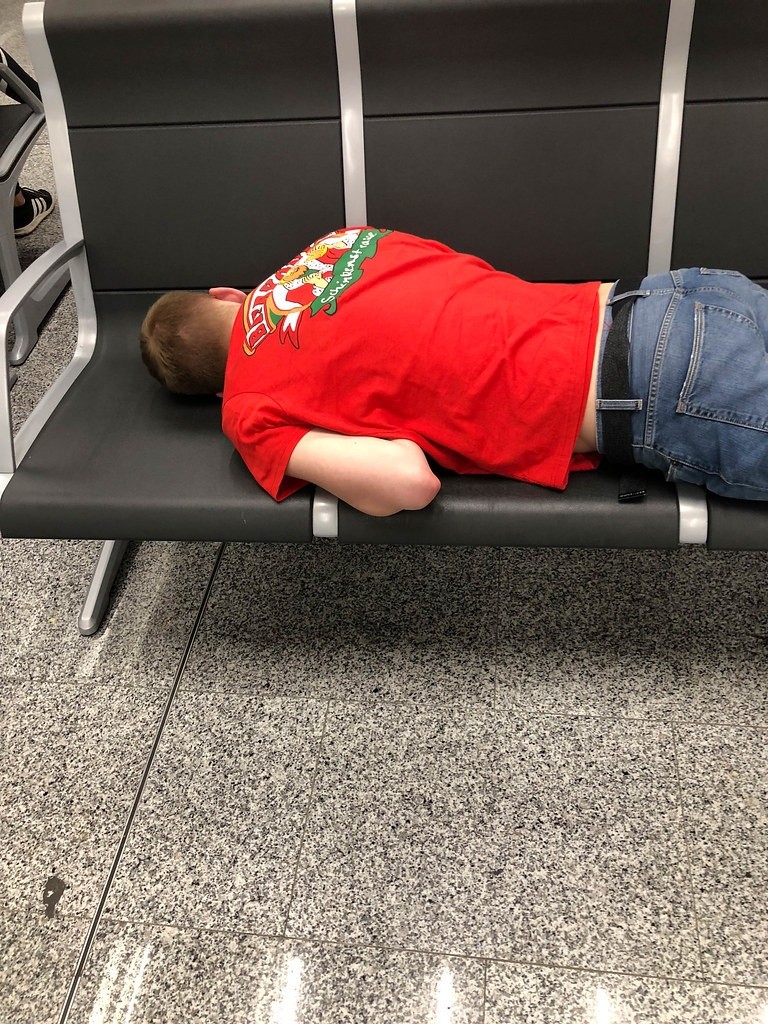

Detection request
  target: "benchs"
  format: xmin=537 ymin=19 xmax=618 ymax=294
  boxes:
xmin=0 ymin=0 xmax=768 ymax=635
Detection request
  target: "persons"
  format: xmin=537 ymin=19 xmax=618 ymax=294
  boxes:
xmin=139 ymin=226 xmax=768 ymax=517
xmin=0 ymin=47 xmax=54 ymax=239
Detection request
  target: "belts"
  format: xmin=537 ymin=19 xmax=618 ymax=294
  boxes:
xmin=601 ymin=275 xmax=646 ymax=499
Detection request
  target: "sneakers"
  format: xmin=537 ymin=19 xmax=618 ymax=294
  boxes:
xmin=14 ymin=187 xmax=54 ymax=235
